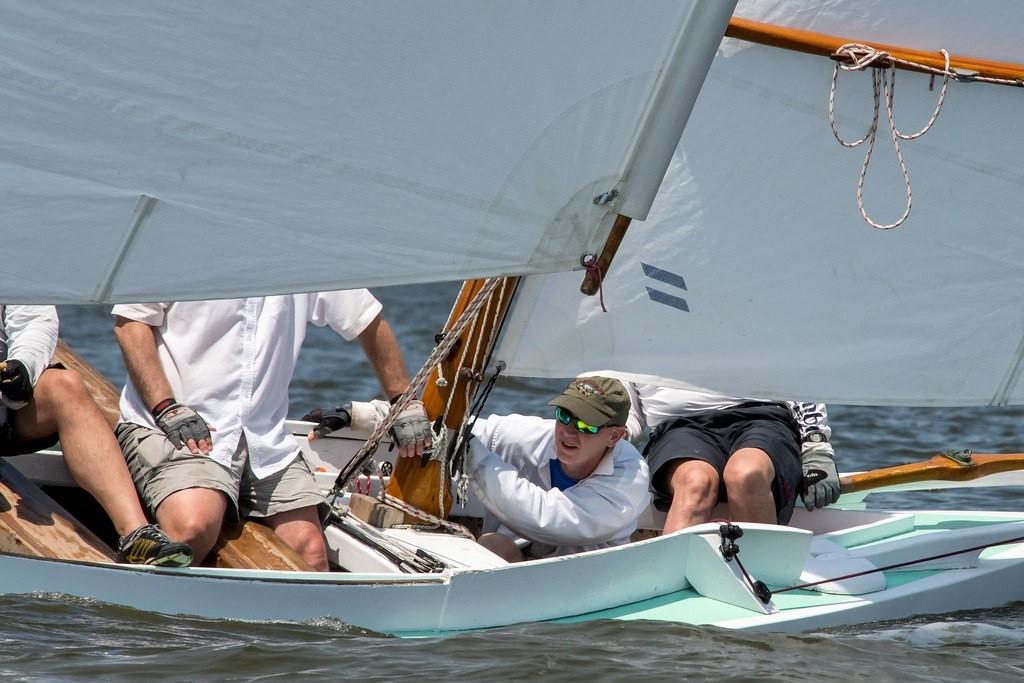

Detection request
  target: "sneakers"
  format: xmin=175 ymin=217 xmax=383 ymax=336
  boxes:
xmin=117 ymin=524 xmax=194 ymax=567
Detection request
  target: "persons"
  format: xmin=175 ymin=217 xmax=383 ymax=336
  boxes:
xmin=294 ymin=377 xmax=651 ymax=563
xmin=619 ymin=381 xmax=840 ymax=534
xmin=111 ymin=288 xmax=432 ymax=571
xmin=0 ymin=304 xmax=193 ymax=567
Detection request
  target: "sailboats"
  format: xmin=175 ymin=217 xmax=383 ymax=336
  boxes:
xmin=0 ymin=1 xmax=1023 ymax=639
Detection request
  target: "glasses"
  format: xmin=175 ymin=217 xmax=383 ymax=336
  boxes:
xmin=555 ymin=405 xmax=610 ymax=434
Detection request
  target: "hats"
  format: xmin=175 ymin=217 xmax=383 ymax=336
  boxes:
xmin=546 ymin=376 xmax=631 ymax=427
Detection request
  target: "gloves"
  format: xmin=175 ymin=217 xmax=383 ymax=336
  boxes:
xmin=801 ymin=450 xmax=841 ymax=512
